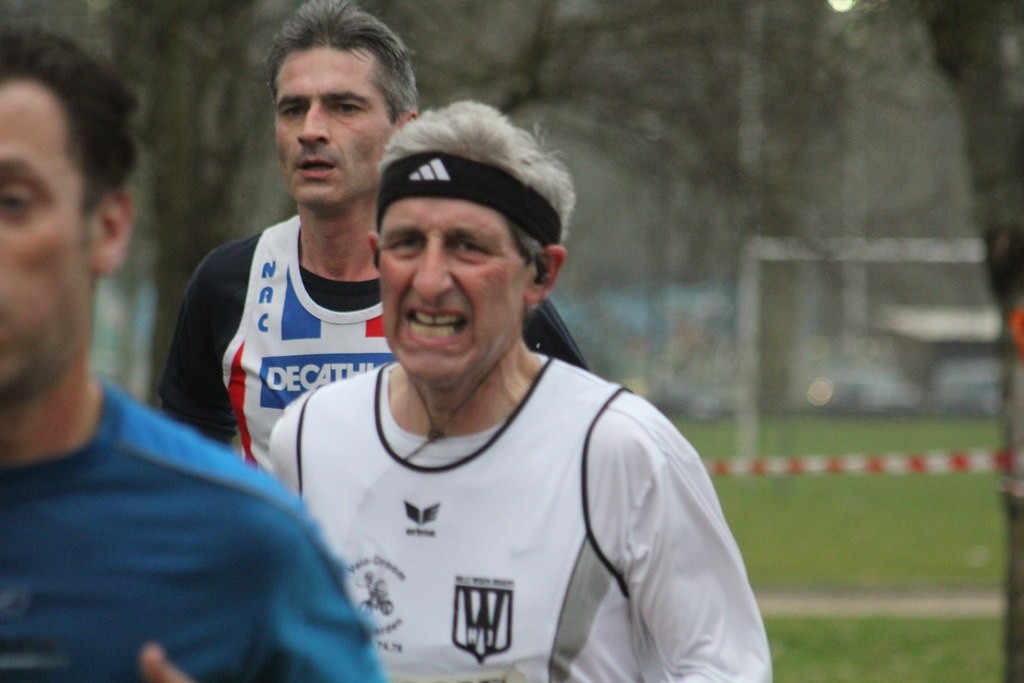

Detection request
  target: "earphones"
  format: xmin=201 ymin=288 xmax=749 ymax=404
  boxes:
xmin=534 ymin=259 xmax=548 ymax=285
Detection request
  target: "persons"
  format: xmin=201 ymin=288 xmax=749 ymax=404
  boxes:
xmin=0 ymin=19 xmax=389 ymax=683
xmin=270 ymin=99 xmax=774 ymax=683
xmin=157 ymin=1 xmax=591 ymax=476
